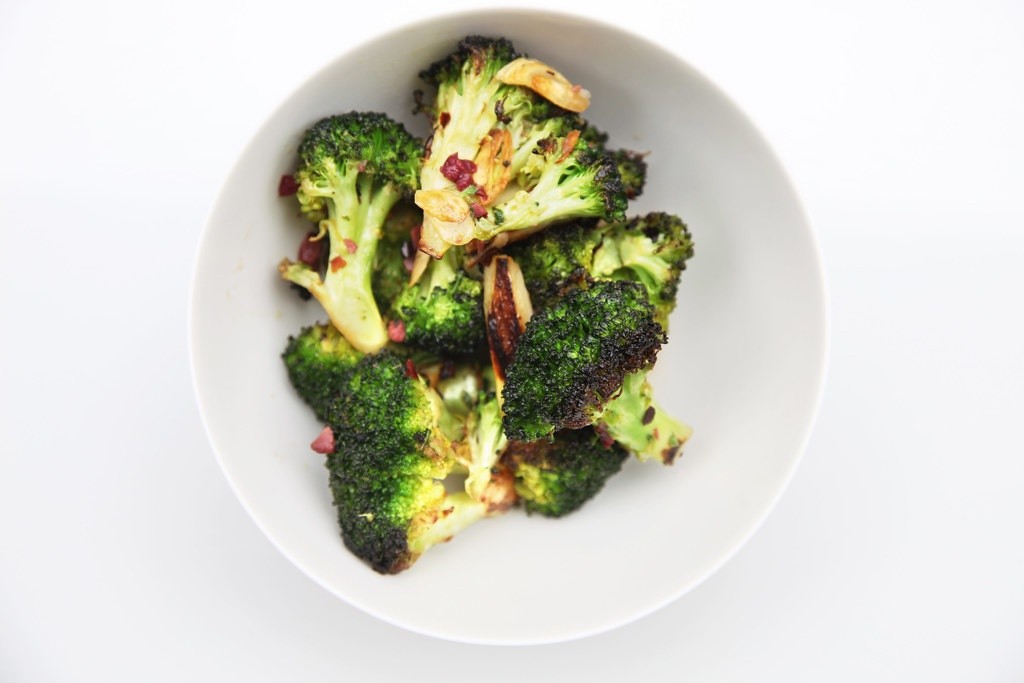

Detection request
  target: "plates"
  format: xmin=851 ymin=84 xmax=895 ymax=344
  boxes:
xmin=187 ymin=8 xmax=827 ymax=643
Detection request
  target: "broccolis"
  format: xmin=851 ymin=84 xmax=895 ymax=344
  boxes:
xmin=279 ymin=35 xmax=693 ymax=575
